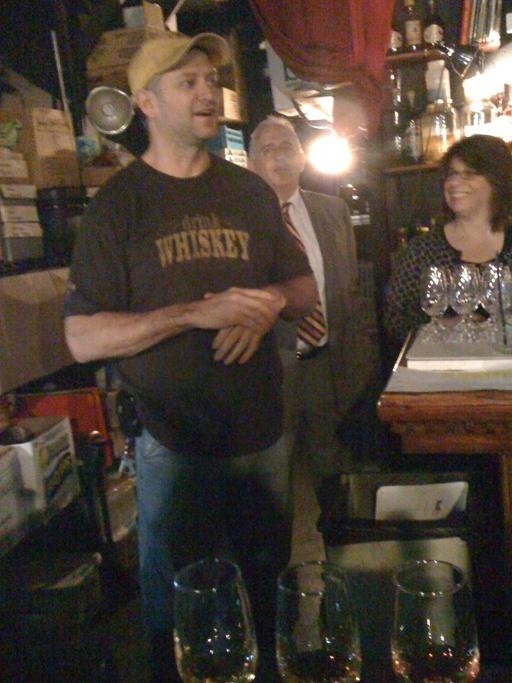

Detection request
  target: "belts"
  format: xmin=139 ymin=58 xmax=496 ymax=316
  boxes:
xmin=294 ymin=342 xmax=328 ymax=361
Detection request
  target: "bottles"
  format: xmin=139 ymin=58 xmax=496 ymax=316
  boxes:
xmin=390 ymin=225 xmax=411 ymax=272
xmin=338 ymin=183 xmax=372 ymax=251
xmin=381 ymin=59 xmax=499 ymax=166
xmin=388 ymin=0 xmax=443 ymax=53
xmin=38 ymin=185 xmax=100 ymax=268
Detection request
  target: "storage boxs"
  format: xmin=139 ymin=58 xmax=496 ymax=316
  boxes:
xmin=7 ymin=417 xmax=81 ymax=514
xmin=0 ymin=447 xmax=30 ymax=554
xmin=1 ymin=2 xmax=251 ymax=266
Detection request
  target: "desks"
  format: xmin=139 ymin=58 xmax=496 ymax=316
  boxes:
xmin=378 ymin=320 xmax=511 ymax=558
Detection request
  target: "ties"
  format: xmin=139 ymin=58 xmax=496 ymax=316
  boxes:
xmin=280 ymin=201 xmax=328 ymax=348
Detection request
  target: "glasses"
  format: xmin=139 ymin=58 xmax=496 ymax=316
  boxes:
xmin=253 ymin=145 xmax=303 ymax=157
xmin=443 ymin=169 xmax=484 ymax=182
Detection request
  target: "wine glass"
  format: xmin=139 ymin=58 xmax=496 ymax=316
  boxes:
xmin=424 ymin=260 xmax=511 ymax=346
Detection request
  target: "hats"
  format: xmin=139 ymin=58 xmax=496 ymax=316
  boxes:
xmin=128 ymin=33 xmax=232 ymax=93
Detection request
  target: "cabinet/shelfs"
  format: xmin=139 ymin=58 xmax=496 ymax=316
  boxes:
xmin=383 ymin=47 xmax=451 ymax=246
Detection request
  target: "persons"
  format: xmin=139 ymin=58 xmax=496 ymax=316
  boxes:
xmin=243 ymin=109 xmax=362 ymax=553
xmin=58 ymin=32 xmax=319 ymax=671
xmin=379 ymin=132 xmax=512 ymax=340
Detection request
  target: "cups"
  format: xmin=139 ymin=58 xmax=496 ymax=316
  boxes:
xmin=388 ymin=557 xmax=481 ymax=680
xmin=275 ymin=557 xmax=364 ymax=682
xmin=171 ymin=554 xmax=258 ymax=680
